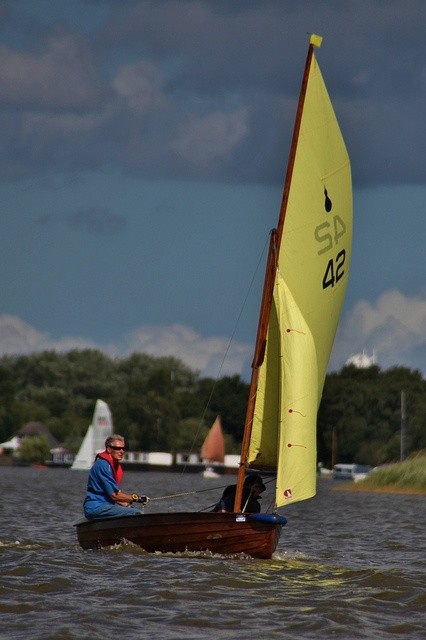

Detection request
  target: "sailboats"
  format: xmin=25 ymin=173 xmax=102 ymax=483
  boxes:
xmin=74 ymin=33 xmax=354 ymax=560
xmin=200 ymin=415 xmax=225 ymax=479
xmin=70 ymin=399 xmax=113 ymax=470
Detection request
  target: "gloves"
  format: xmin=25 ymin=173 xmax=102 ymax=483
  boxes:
xmin=131 ymin=494 xmax=150 ymax=505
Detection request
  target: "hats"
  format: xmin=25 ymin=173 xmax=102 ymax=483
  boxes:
xmin=242 ymin=475 xmax=264 ymax=493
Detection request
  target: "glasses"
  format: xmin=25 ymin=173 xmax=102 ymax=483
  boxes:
xmin=107 ymin=445 xmax=125 ymax=450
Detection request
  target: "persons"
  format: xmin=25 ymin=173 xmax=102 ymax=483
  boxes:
xmin=83 ymin=434 xmax=152 ymax=521
xmin=210 ymin=475 xmax=263 ymax=514
